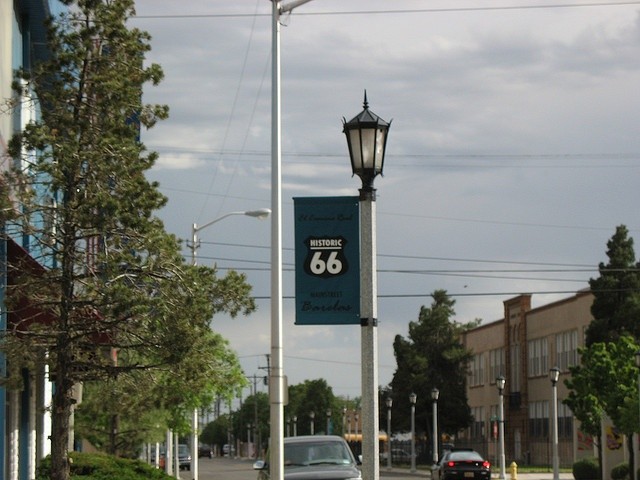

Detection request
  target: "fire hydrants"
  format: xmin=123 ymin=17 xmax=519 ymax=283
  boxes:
xmin=510 ymin=461 xmax=518 ymax=480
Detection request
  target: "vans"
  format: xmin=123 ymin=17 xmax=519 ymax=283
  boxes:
xmin=222 ymin=443 xmax=234 ymax=453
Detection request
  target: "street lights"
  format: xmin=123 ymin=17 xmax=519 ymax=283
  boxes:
xmin=292 ymin=415 xmax=298 ymax=437
xmin=325 ymin=407 xmax=332 ymax=435
xmin=385 ymin=396 xmax=393 ymax=468
xmin=286 ymin=417 xmax=291 ymax=437
xmin=409 ymin=392 xmax=418 ymax=472
xmin=354 ymin=412 xmax=359 ymax=443
xmin=496 ymin=375 xmax=507 ymax=480
xmin=309 ymin=410 xmax=316 ymax=435
xmin=246 ymin=423 xmax=251 ymax=458
xmin=342 ymin=89 xmax=393 ymax=475
xmin=189 ymin=209 xmax=270 ymax=480
xmin=430 ymin=387 xmax=440 ymax=480
xmin=347 ymin=415 xmax=351 ymax=443
xmin=549 ymin=366 xmax=561 ymax=480
xmin=343 ymin=405 xmax=347 ymax=436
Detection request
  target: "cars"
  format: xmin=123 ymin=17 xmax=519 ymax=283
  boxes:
xmin=438 ymin=448 xmax=491 ymax=480
xmin=146 ymin=446 xmax=166 ymax=470
xmin=379 ymin=449 xmax=410 ymax=464
xmin=253 ymin=434 xmax=362 ymax=480
xmin=172 ymin=444 xmax=191 ymax=470
xmin=199 ymin=446 xmax=211 ymax=459
